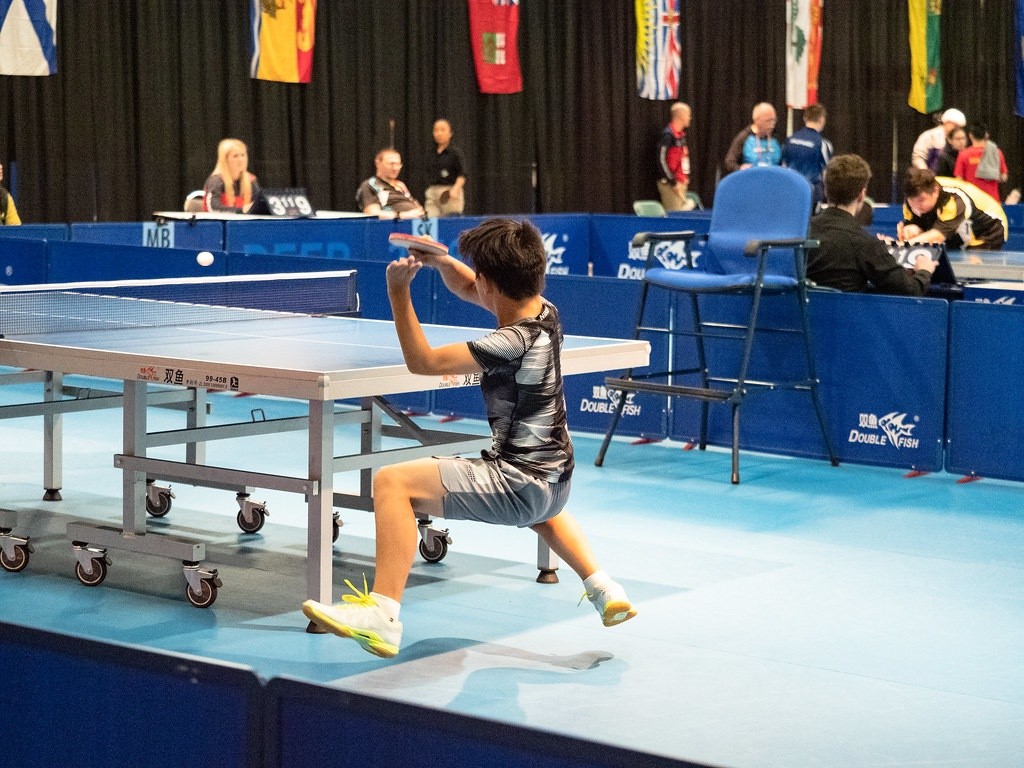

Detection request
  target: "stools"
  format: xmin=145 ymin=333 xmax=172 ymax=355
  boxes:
xmin=596 ymin=167 xmax=839 ymax=484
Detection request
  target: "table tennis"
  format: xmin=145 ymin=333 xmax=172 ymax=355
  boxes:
xmin=196 ymin=252 xmax=214 ymax=267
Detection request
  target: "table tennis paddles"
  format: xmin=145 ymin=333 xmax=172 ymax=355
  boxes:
xmin=388 ymin=233 xmax=448 ymax=257
xmin=439 ymin=190 xmax=451 ymax=205
xmin=852 ymin=199 xmax=875 ymax=225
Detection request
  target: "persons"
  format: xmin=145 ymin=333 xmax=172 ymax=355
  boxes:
xmin=806 ymin=154 xmax=939 ymax=296
xmin=877 ymin=169 xmax=1008 ymax=250
xmin=725 ymin=102 xmax=781 ymax=173
xmin=657 ymin=102 xmax=692 ymax=212
xmin=937 ymin=128 xmax=967 ymax=176
xmin=202 ymin=139 xmax=262 ymax=213
xmin=424 ymin=118 xmax=468 ymax=217
xmin=953 ymin=120 xmax=1008 ymax=199
xmin=302 ymin=219 xmax=638 ymax=659
xmin=358 ymin=150 xmax=426 ymax=220
xmin=912 ymin=108 xmax=967 ymax=172
xmin=778 ymin=103 xmax=834 ymax=206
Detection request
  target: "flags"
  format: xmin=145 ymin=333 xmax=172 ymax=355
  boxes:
xmin=249 ymin=0 xmax=316 ymax=85
xmin=468 ymin=0 xmax=523 ymax=93
xmin=908 ymin=0 xmax=944 ymax=113
xmin=784 ymin=0 xmax=824 ymax=110
xmin=0 ymin=0 xmax=58 ymax=76
xmin=634 ymin=0 xmax=681 ymax=101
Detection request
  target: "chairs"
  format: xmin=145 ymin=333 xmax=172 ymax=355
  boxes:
xmin=185 ymin=190 xmax=206 ymax=211
xmin=685 ymin=191 xmax=705 ymax=212
xmin=632 ymin=200 xmax=668 ymax=219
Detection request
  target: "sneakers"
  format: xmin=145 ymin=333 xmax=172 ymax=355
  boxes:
xmin=301 ymin=574 xmax=402 ymax=657
xmin=577 ymin=581 xmax=635 ymax=629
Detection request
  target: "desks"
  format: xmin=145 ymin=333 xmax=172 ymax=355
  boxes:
xmin=150 ymin=208 xmax=382 ymax=224
xmin=864 ymin=244 xmax=1024 ymax=295
xmin=1 ymin=284 xmax=652 ymax=633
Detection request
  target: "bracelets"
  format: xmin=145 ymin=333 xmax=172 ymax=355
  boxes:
xmin=397 ymin=211 xmax=400 ymax=218
